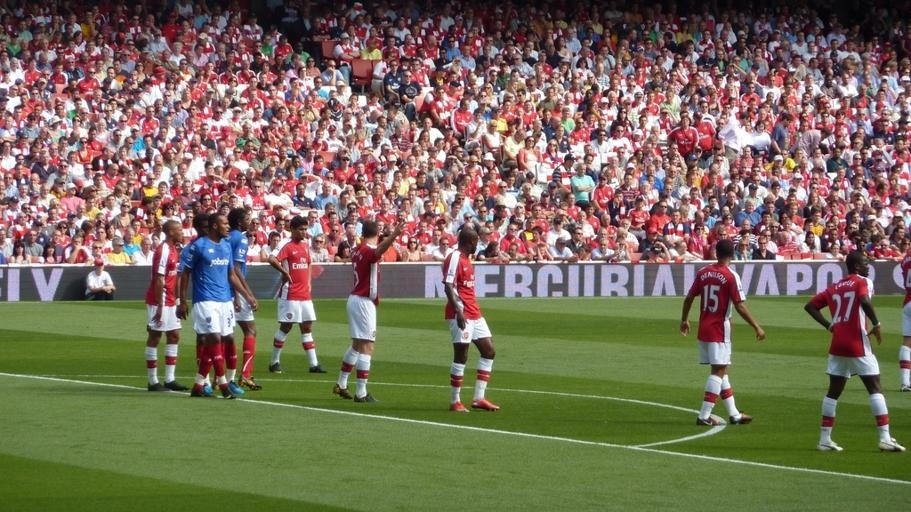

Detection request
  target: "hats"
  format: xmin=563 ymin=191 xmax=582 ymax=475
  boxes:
xmin=483 ymin=3 xmax=911 ymax=257
xmin=1 ymin=2 xmax=482 ymax=259
xmin=94 ymin=259 xmax=103 ymax=266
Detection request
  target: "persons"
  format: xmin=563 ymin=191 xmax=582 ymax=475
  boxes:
xmin=331 ymin=217 xmax=407 ymax=402
xmin=679 ymin=238 xmax=766 ymax=425
xmin=2 ymin=2 xmax=910 ymax=266
xmin=144 ymin=220 xmax=190 ymax=392
xmin=804 ymin=251 xmax=906 ymax=452
xmin=84 ymin=258 xmax=115 ymax=301
xmin=268 ymin=215 xmax=327 ymax=375
xmin=899 ymin=249 xmax=911 ymax=392
xmin=179 ymin=208 xmax=261 ymax=400
xmin=441 ymin=227 xmax=500 ymax=412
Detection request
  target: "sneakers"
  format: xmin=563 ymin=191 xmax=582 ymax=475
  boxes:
xmin=354 ymin=393 xmax=375 ymax=401
xmin=472 ymin=399 xmax=499 ymax=411
xmin=148 ymin=362 xmax=327 ymax=399
xmin=696 ymin=417 xmax=718 ymax=425
xmin=450 ymin=402 xmax=470 ymax=412
xmin=333 ymin=383 xmax=353 ymax=399
xmin=817 ymin=441 xmax=843 ymax=451
xmin=899 ymin=384 xmax=911 ymax=392
xmin=879 ymin=437 xmax=906 ymax=451
xmin=730 ymin=411 xmax=752 ymax=423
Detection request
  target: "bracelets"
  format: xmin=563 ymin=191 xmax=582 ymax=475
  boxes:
xmin=156 ymin=303 xmax=164 ymax=308
xmin=828 ymin=323 xmax=833 ymax=330
xmin=179 ymin=297 xmax=186 ymax=304
xmin=680 ymin=318 xmax=686 ymax=322
xmin=872 ymin=322 xmax=881 ymax=328
